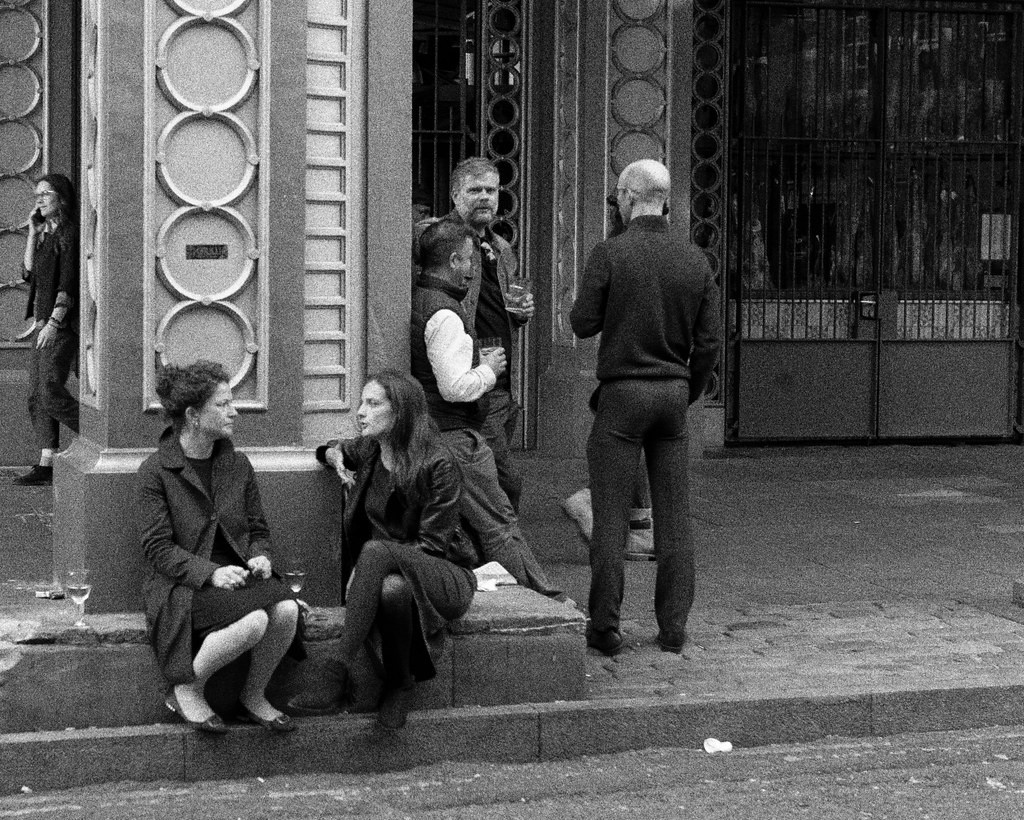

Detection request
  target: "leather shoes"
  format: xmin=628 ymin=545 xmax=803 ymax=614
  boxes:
xmin=586 ymin=629 xmax=622 ymax=656
xmin=656 ymin=632 xmax=685 ymax=654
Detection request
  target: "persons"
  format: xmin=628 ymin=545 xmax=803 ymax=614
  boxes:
xmin=132 ymin=361 xmax=308 ymax=732
xmin=567 ymin=157 xmax=725 ymax=656
xmin=405 ymin=156 xmax=671 ymax=606
xmin=12 ymin=173 xmax=81 ymax=484
xmin=286 ymin=368 xmax=476 ymax=731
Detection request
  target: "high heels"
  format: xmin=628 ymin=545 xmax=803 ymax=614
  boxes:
xmin=238 ymin=693 xmax=294 ymax=732
xmin=165 ymin=690 xmax=227 ymax=735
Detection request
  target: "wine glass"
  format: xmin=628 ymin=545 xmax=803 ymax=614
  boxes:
xmin=66 ymin=569 xmax=93 ymax=629
xmin=285 ymin=569 xmax=306 ymax=599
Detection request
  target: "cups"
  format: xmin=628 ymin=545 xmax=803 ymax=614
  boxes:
xmin=476 ymin=337 xmax=502 ymax=356
xmin=505 ymin=276 xmax=533 ymax=314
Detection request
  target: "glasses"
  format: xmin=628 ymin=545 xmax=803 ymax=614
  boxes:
xmin=33 ymin=191 xmax=56 ymax=199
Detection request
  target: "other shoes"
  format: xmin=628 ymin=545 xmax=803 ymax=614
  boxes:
xmin=379 ymin=674 xmax=413 ymax=730
xmin=543 ymin=591 xmax=563 ymax=600
xmin=290 ymin=658 xmax=354 ymax=714
xmin=12 ymin=466 xmax=54 ymax=485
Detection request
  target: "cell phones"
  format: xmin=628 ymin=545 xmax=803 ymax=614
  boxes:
xmin=34 ymin=209 xmax=46 ymax=223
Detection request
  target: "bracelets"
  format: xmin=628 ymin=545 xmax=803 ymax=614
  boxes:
xmin=51 ymin=317 xmax=63 ymax=328
